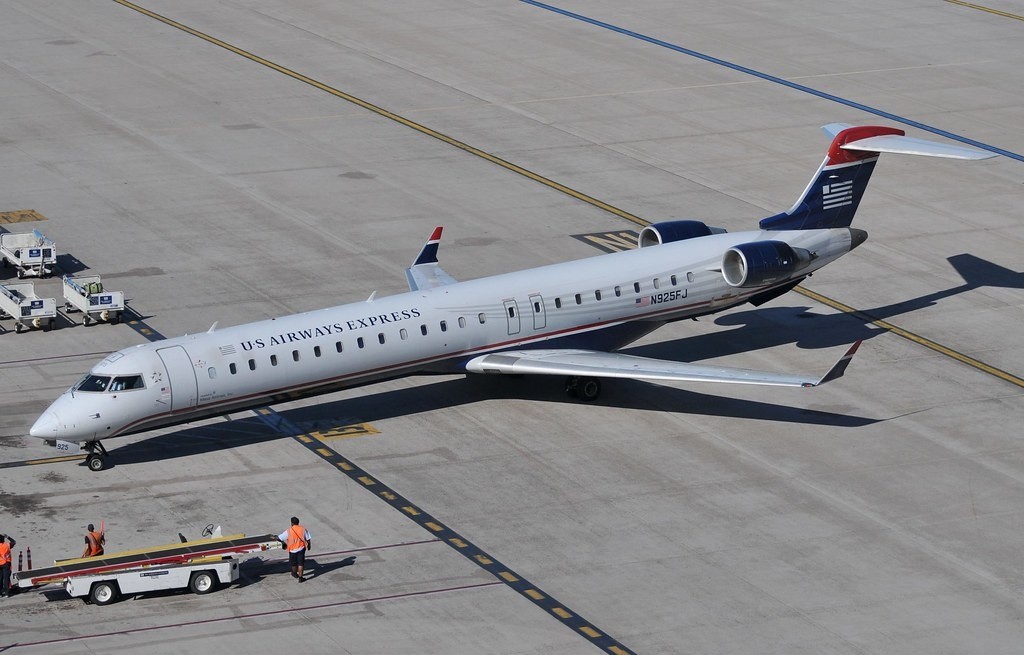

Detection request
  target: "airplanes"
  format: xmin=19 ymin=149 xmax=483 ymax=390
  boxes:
xmin=27 ymin=116 xmax=999 ymax=471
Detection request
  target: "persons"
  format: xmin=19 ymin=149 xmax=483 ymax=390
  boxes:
xmin=0 ymin=534 xmax=16 ymax=598
xmin=82 ymin=524 xmax=105 ymax=558
xmin=270 ymin=517 xmax=312 ymax=583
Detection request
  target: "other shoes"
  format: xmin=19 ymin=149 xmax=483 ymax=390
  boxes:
xmin=5 ymin=594 xmax=9 ymax=598
xmin=291 ymin=571 xmax=298 ymax=578
xmin=0 ymin=595 xmax=3 ymax=598
xmin=299 ymin=577 xmax=306 ymax=583
